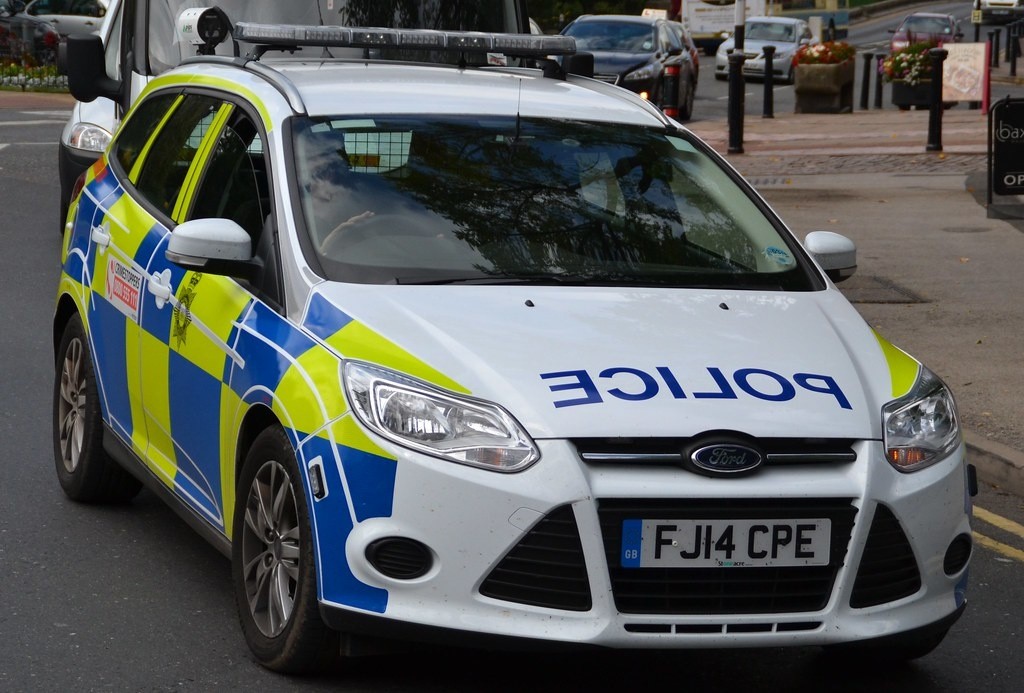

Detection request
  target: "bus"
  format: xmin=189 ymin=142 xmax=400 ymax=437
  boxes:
xmin=681 ymin=1 xmax=852 ymax=56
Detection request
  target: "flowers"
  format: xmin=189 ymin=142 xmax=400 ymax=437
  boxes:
xmin=878 ymin=28 xmax=949 ymax=87
xmin=791 ymin=41 xmax=857 ymax=64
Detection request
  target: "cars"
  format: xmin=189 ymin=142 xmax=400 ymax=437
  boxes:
xmin=669 ymin=20 xmax=701 ymax=92
xmin=886 ymin=9 xmax=965 ymax=53
xmin=535 ymin=15 xmax=696 ymax=126
xmin=970 ymin=0 xmax=1024 ymax=25
xmin=52 ymin=4 xmax=981 ymax=676
xmin=713 ymin=15 xmax=820 ymax=84
xmin=1 ymin=0 xmax=111 ymax=70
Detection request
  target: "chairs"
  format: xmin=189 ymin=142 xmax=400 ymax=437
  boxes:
xmin=249 ymin=210 xmax=280 ymax=302
xmin=779 ymin=31 xmax=793 ymax=41
xmin=471 ymin=175 xmax=612 ymax=264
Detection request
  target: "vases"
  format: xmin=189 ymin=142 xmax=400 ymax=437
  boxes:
xmin=892 ymin=72 xmax=958 ymax=111
xmin=794 ymin=56 xmax=855 ymax=114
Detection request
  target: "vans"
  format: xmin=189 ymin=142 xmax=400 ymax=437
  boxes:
xmin=56 ymin=0 xmax=532 ymax=241
